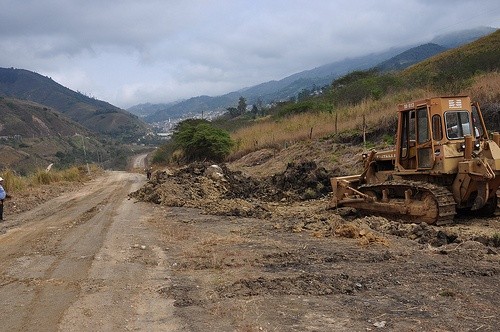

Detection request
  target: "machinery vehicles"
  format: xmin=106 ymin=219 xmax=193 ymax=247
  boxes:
xmin=325 ymin=96 xmax=500 ymax=227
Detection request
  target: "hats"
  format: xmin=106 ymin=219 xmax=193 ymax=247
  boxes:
xmin=0 ymin=177 xmax=4 ymax=181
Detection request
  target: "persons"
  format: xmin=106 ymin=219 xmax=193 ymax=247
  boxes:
xmin=447 ymin=118 xmax=481 ymax=149
xmin=144 ymin=163 xmax=154 ymax=179
xmin=0 ymin=177 xmax=6 ymax=222
